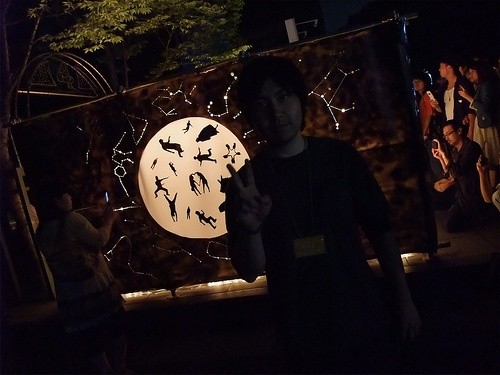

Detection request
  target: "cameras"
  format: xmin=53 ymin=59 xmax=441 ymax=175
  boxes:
xmin=432 ymin=141 xmax=438 ymax=149
xmin=104 ymin=191 xmax=111 ymax=208
xmin=481 ymin=157 xmax=488 ymax=167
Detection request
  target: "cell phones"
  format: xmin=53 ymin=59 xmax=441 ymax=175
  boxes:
xmin=426 ymin=91 xmax=439 ymax=104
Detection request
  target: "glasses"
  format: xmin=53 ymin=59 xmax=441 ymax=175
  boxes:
xmin=442 ymin=127 xmax=459 ymax=138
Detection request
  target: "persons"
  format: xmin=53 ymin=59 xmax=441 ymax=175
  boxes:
xmin=32 ymin=185 xmax=134 ymax=375
xmin=412 ymin=57 xmax=500 ymax=233
xmin=225 ymin=55 xmax=423 ymax=375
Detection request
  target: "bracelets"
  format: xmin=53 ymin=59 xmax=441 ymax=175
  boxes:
xmin=238 ymin=223 xmax=264 ymax=234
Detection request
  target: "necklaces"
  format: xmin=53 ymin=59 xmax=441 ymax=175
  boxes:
xmin=268 ymin=134 xmax=327 ymax=258
